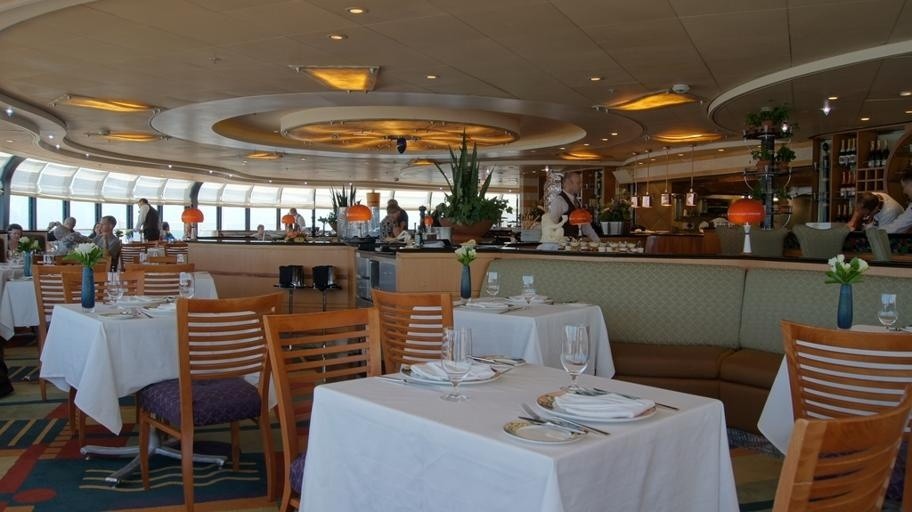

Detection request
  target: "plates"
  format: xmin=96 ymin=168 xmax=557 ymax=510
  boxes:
xmin=94 ymin=308 xmax=176 ymax=319
xmin=503 ymin=418 xmax=587 ymax=445
xmin=535 ymin=389 xmax=657 ymax=422
xmin=456 ymin=295 xmax=552 ymax=314
xmin=901 ymin=325 xmax=912 ymax=334
xmin=9 ymin=265 xmax=24 ymax=269
xmin=400 ymin=360 xmax=502 ymax=384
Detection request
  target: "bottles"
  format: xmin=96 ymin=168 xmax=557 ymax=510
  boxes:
xmin=414 ymin=225 xmax=423 ymax=248
xmin=836 ymin=138 xmax=856 ymax=223
xmin=868 ymin=139 xmax=890 ymax=168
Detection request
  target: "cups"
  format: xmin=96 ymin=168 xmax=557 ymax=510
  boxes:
xmin=179 ymin=272 xmax=195 ymax=299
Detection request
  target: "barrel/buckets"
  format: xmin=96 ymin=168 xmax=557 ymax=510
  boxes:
xmin=280 ymin=265 xmax=304 ymax=287
xmin=600 ymin=220 xmax=628 ymax=234
xmin=314 ymin=264 xmax=336 ymax=285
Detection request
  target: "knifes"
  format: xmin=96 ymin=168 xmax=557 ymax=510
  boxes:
xmin=375 ymin=376 xmax=432 ymax=387
xmin=593 ymin=387 xmax=680 ymax=411
xmin=517 ymin=415 xmax=588 ymax=434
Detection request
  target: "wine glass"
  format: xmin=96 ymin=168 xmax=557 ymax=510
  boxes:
xmin=878 ymin=293 xmax=898 ymax=332
xmin=439 ymin=327 xmax=474 ymax=401
xmin=521 ymin=273 xmax=537 ymax=310
xmin=561 ymin=322 xmax=590 ymax=389
xmin=486 ymin=271 xmax=500 ymax=302
xmin=106 ymin=271 xmax=124 ymax=312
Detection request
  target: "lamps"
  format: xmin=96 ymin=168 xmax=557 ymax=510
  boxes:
xmin=630 ymin=145 xmax=697 ymax=209
xmin=281 ymin=213 xmax=296 ymax=232
xmin=181 ymin=206 xmax=204 ymax=241
xmin=344 ymin=201 xmax=372 ymax=240
xmin=728 ymin=199 xmax=765 ymax=254
xmin=568 ymin=210 xmax=593 ymax=238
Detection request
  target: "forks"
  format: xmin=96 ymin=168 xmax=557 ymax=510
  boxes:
xmin=519 ymin=403 xmax=609 ymax=435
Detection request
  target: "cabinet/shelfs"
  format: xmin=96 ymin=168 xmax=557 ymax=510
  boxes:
xmin=538 ymin=167 xmax=616 ymax=207
xmin=806 ymin=123 xmax=912 ymax=223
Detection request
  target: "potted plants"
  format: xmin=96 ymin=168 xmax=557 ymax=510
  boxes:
xmin=743 ymin=101 xmax=801 ymax=212
xmin=432 ymin=128 xmax=513 ymax=246
xmin=600 ymin=204 xmax=629 ymax=234
xmin=317 ymin=182 xmax=362 ymax=233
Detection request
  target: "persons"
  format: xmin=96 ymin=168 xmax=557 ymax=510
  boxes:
xmin=252 ymin=224 xmax=266 ymax=241
xmin=550 ymin=171 xmax=601 ymax=243
xmin=289 ymin=208 xmax=306 ymax=232
xmin=380 ymin=199 xmax=447 ymax=240
xmin=0 ymin=197 xmax=177 ymax=273
xmin=847 ymin=191 xmax=905 ymax=232
xmin=877 ymin=169 xmax=912 ymax=234
